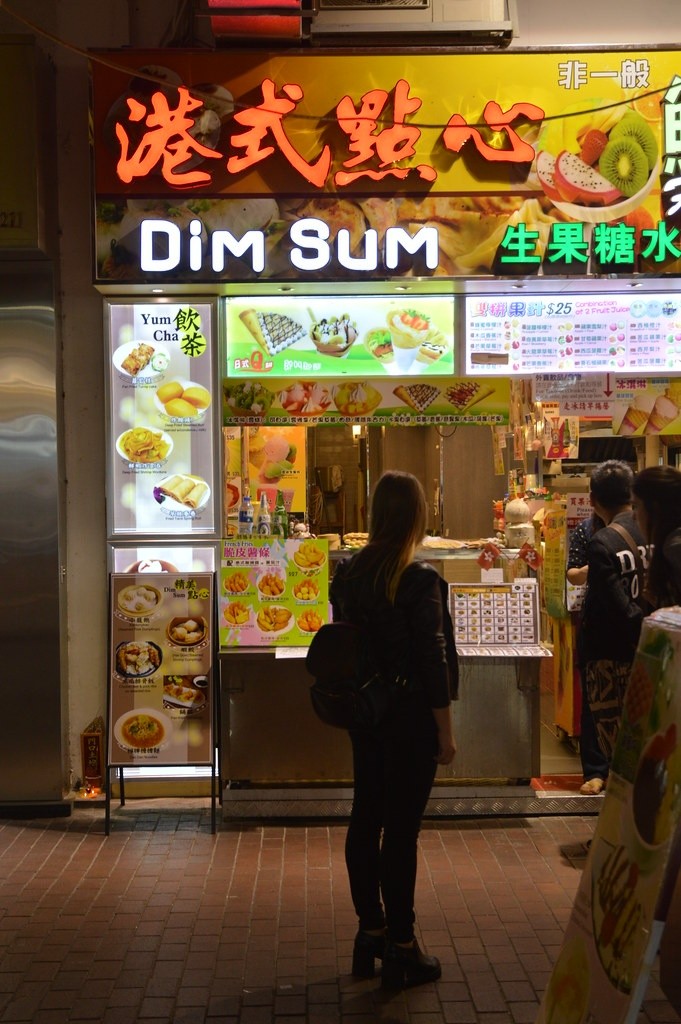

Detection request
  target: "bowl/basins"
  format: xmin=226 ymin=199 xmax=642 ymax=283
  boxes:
xmin=257 ymin=581 xmax=286 ymax=601
xmin=281 ymin=383 xmax=330 ymax=416
xmin=540 ymin=161 xmax=660 ymax=227
xmin=292 ymin=583 xmax=320 ymax=601
xmin=335 ymin=384 xmax=383 ymax=417
xmin=224 ymin=606 xmax=250 ymax=626
xmin=226 ymin=386 xmax=273 ymax=426
xmin=293 ymin=552 xmax=327 ymax=577
xmin=257 ymin=605 xmax=295 ymax=636
xmin=222 ymin=579 xmax=248 ymax=593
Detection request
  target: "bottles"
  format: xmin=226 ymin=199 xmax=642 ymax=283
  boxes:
xmin=564 ymin=419 xmax=571 ymax=447
xmin=274 ymin=489 xmax=289 ymax=538
xmin=551 ymin=417 xmax=560 ymax=447
xmin=239 ymin=496 xmax=254 ymax=533
xmin=258 ymin=491 xmax=272 ymax=534
xmin=571 ymin=420 xmax=576 ymax=443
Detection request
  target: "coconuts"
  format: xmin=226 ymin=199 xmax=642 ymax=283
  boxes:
xmin=504 ymin=499 xmax=530 ymax=523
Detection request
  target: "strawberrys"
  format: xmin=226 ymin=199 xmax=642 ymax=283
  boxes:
xmin=402 ymin=309 xmax=431 ymax=331
xmin=583 ymin=130 xmax=608 ymax=166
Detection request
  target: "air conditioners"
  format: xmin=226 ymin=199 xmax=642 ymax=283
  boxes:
xmin=308 ymin=0 xmax=520 ymax=45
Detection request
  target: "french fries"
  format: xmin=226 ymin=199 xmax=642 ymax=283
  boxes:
xmin=223 ymin=542 xmax=322 ymax=628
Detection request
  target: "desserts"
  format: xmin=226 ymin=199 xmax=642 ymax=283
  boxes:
xmin=418 ymin=329 xmax=447 ymax=359
xmin=392 ymin=380 xmax=497 ymax=413
xmin=278 ymin=381 xmax=383 ymax=418
xmin=238 ymin=309 xmax=308 ymax=356
xmin=387 ymin=307 xmax=430 ymax=349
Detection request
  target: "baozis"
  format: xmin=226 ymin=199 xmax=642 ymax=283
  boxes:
xmin=123 ymin=587 xmax=157 ymax=611
xmin=172 ymin=620 xmax=202 ymax=643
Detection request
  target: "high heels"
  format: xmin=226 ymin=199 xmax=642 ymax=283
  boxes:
xmin=382 ymin=940 xmax=441 ymax=990
xmin=351 ymin=931 xmax=388 ymax=980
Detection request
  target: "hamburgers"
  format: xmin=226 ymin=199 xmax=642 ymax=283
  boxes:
xmin=363 ymin=326 xmax=394 ymax=363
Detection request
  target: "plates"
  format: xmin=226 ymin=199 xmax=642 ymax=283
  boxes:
xmin=297 ymin=615 xmax=324 ymax=633
xmin=164 ymin=686 xmax=205 ymax=708
xmin=113 ymin=340 xmax=169 ymax=379
xmin=154 ymin=474 xmax=210 ymax=511
xmin=115 ymin=640 xmax=163 ymax=677
xmin=114 ymin=708 xmax=174 ymax=750
xmin=116 ymin=426 xmax=173 ymax=463
xmin=155 ymin=380 xmax=211 ymax=418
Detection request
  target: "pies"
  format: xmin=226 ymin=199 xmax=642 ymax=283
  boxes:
xmin=159 ymin=476 xmax=208 ymax=509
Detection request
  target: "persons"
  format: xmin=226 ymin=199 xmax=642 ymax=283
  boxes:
xmin=566 ymin=459 xmax=681 ymax=796
xmin=327 ymin=469 xmax=461 ymax=997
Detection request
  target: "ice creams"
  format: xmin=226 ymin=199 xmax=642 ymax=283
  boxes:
xmin=616 ymin=395 xmax=680 ymax=435
xmin=305 ymin=306 xmax=358 ymax=359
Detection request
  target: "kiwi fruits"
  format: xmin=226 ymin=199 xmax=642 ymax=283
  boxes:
xmin=599 ymin=110 xmax=660 ymax=196
xmin=224 ymin=384 xmax=274 ymax=411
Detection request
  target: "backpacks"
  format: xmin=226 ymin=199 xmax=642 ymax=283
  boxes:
xmin=307 ymin=561 xmax=423 ymax=728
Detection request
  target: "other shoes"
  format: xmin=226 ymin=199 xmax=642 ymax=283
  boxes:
xmin=580 ymin=777 xmax=605 ymax=794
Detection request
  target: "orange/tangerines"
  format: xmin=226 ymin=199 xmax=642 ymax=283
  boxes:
xmin=632 ymin=89 xmax=666 ymax=122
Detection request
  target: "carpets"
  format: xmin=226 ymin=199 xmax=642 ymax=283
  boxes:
xmin=529 ymin=773 xmax=585 ymax=790
